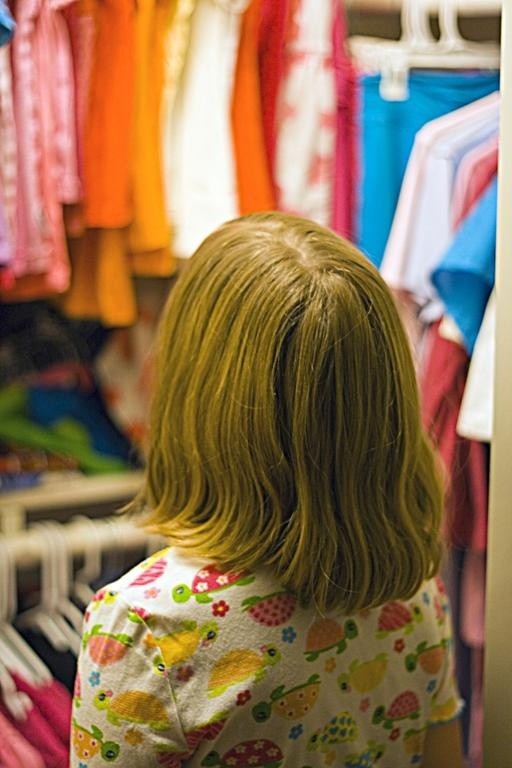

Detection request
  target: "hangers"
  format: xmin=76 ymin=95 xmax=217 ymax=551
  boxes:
xmin=1 ymin=516 xmax=170 ymax=722
xmin=342 ymin=0 xmax=503 ymax=103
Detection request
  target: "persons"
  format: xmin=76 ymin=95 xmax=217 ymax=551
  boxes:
xmin=71 ymin=211 xmax=468 ymax=768
